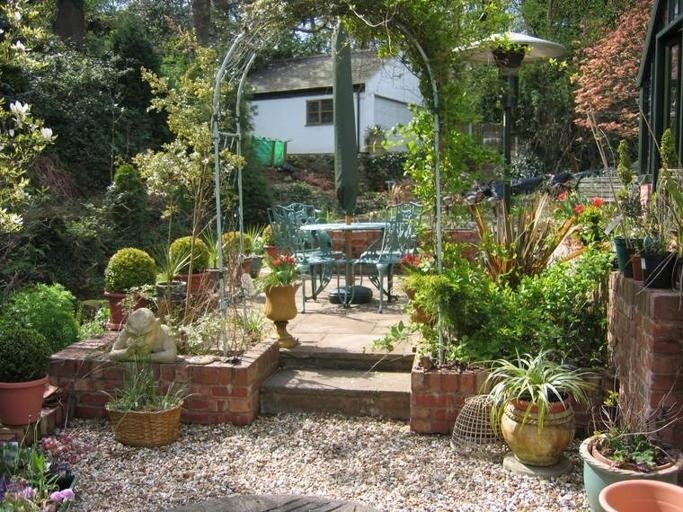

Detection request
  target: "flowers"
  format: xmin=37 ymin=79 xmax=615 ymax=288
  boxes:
xmin=0 ymin=459 xmax=74 ymax=512
xmin=259 ymin=251 xmax=299 ymax=287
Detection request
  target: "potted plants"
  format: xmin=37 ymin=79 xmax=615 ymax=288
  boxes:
xmin=100 ymin=340 xmax=189 ymax=448
xmin=103 ymin=219 xmax=288 ymax=331
xmin=0 ymin=324 xmax=51 ymax=426
xmin=612 ymin=126 xmax=679 ymax=288
xmin=589 ymin=384 xmax=683 ymax=470
xmin=474 ymin=346 xmax=599 ymax=469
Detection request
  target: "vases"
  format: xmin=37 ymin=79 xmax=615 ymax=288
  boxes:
xmin=580 ymin=434 xmax=683 ymax=512
xmin=261 ymin=282 xmax=300 ymax=348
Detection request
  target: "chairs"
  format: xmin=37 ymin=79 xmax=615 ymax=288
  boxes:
xmin=264 ymin=201 xmax=423 ymax=316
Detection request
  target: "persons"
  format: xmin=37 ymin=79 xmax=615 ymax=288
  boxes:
xmin=109 ymin=308 xmax=177 ymax=364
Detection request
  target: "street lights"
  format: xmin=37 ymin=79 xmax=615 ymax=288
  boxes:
xmin=451 ymin=29 xmax=565 ymax=218
